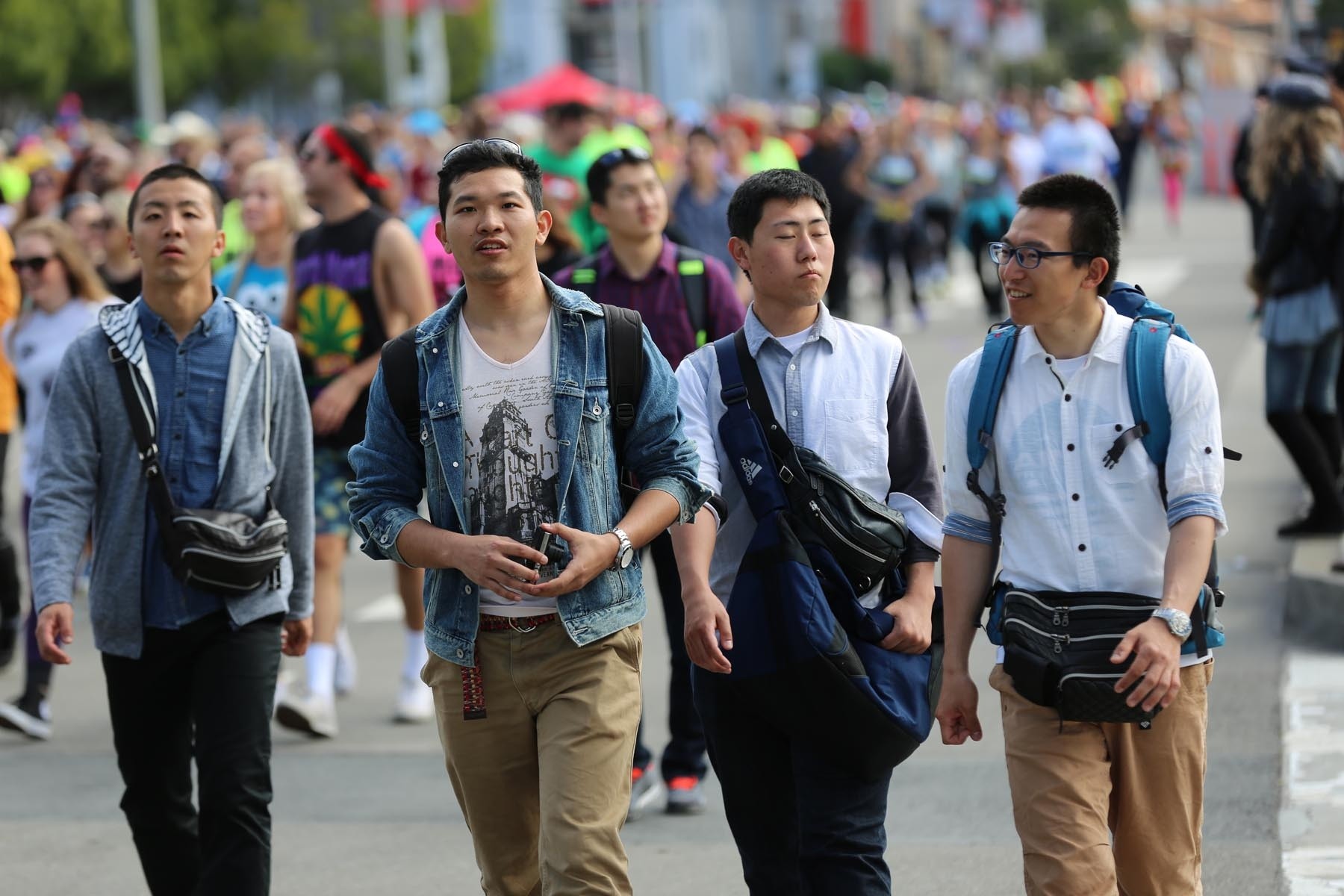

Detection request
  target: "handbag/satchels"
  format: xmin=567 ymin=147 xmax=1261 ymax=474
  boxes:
xmin=775 ymin=447 xmax=907 ymax=597
xmin=159 ymin=503 xmax=289 ymax=600
xmin=719 ymin=518 xmax=944 ymax=769
xmin=989 ymin=582 xmax=1202 ymax=731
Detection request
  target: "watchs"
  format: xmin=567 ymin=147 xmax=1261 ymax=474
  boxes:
xmin=604 ymin=528 xmax=632 ymax=573
xmin=1151 ymin=607 xmax=1193 ymax=644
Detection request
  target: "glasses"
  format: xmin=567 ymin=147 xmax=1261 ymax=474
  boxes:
xmin=596 ymin=147 xmax=652 ymax=168
xmin=99 ymin=216 xmax=121 ymax=229
xmin=988 ymin=241 xmax=1098 ymax=269
xmin=10 ymin=254 xmax=61 ymax=272
xmin=442 ymin=137 xmax=522 ymax=167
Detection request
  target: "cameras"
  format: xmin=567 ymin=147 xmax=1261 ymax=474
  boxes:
xmin=524 ymin=526 xmax=563 ymax=585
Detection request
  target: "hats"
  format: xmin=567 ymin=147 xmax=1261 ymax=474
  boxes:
xmin=1257 ymin=74 xmax=1334 ymax=108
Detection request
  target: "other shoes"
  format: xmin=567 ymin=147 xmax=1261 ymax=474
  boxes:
xmin=335 ymin=643 xmax=356 ymax=690
xmin=0 ymin=688 xmax=53 ymax=741
xmin=664 ymin=774 xmax=705 ymax=814
xmin=1275 ymin=514 xmax=1344 ymax=540
xmin=274 ymin=696 xmax=341 ymax=740
xmin=390 ymin=684 xmax=433 ymax=722
xmin=627 ymin=758 xmax=660 ymax=818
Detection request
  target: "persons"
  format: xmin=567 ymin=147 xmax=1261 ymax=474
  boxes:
xmin=29 ymin=163 xmax=314 ymax=896
xmin=272 ymin=123 xmax=438 ymax=742
xmin=937 ymin=174 xmax=1228 ymax=896
xmin=664 ymin=167 xmax=942 ymax=896
xmin=1230 ymin=3 xmax=1342 ymax=548
xmin=0 ymin=92 xmax=1190 ymax=739
xmin=344 ymin=141 xmax=700 ymax=896
xmin=554 ymin=149 xmax=742 ymax=822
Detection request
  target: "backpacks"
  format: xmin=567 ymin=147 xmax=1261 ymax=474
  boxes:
xmin=965 ymin=277 xmax=1225 ymax=608
